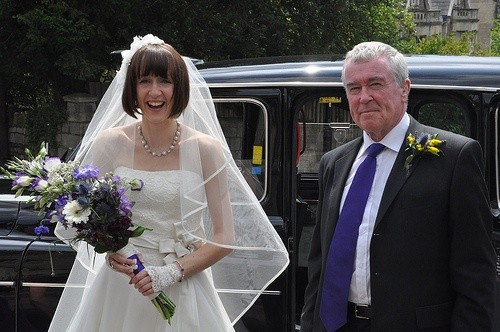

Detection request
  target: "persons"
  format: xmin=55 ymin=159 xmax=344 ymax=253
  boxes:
xmin=300 ymin=41 xmax=497 ymax=332
xmin=47 ymin=34 xmax=290 ymax=332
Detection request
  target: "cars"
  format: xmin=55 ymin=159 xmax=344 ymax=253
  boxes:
xmin=0 ymin=54 xmax=500 ymax=332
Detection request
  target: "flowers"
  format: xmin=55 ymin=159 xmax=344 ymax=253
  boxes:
xmin=0 ymin=141 xmax=176 ymax=326
xmin=405 ymin=131 xmax=446 ymax=169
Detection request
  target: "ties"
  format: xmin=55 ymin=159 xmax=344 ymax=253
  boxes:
xmin=318 ymin=143 xmax=386 ymax=332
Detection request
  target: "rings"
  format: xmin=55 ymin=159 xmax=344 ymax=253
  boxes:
xmin=110 ymin=261 xmax=115 ymax=269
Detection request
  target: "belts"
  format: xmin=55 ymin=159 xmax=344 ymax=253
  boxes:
xmin=347 ymin=301 xmax=372 ymax=320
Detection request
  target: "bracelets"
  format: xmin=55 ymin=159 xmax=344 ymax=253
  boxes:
xmin=173 ymin=259 xmax=184 ymax=282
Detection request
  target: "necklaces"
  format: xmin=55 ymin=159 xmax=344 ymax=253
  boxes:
xmin=138 ymin=119 xmax=180 ymax=157
